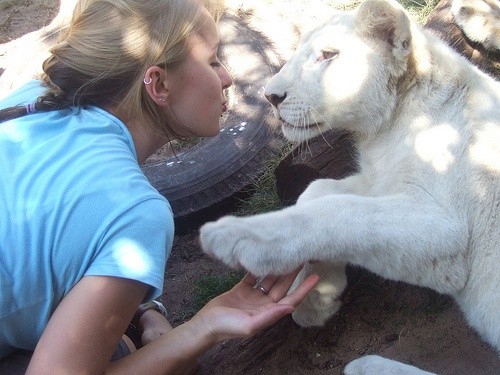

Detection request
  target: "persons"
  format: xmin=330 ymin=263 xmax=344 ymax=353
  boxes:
xmin=0 ymin=0 xmax=321 ymax=375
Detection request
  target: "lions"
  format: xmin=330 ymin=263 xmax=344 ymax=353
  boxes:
xmin=199 ymin=0 xmax=499 ymax=375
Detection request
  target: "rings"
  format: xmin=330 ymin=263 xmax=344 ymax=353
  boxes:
xmin=260 ymin=286 xmax=267 ymax=295
